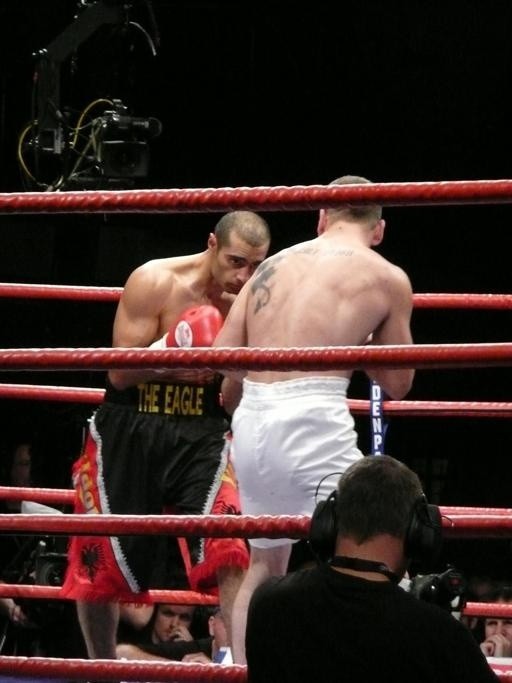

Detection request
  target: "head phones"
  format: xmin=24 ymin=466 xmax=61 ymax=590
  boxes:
xmin=308 ymin=471 xmax=443 ymax=559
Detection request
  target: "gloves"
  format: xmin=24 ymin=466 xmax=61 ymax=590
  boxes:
xmin=149 ymin=304 xmax=223 ymax=348
